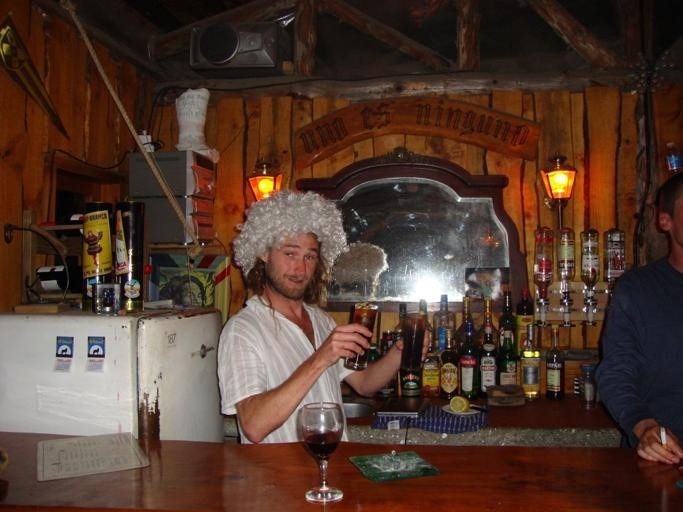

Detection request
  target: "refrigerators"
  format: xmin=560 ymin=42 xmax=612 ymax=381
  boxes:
xmin=1 ymin=431 xmax=225 ymax=511
xmin=0 ymin=308 xmax=226 ymax=445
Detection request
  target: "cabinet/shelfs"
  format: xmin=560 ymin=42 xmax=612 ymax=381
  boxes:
xmin=128 ymin=149 xmax=216 ymax=244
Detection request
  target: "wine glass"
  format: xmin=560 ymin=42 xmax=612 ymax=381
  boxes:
xmin=297 ymin=401 xmax=346 ymax=504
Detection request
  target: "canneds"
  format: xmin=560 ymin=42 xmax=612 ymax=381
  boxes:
xmin=93 ymin=283 xmax=121 ymax=314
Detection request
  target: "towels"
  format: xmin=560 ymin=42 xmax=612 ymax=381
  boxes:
xmin=371 ymin=404 xmax=491 ymax=434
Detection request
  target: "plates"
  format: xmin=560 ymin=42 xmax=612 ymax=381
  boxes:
xmin=441 ymin=404 xmax=482 ymax=415
xmin=486 ymin=398 xmax=526 ymax=408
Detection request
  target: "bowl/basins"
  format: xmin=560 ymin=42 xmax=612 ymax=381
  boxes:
xmin=483 ymin=384 xmax=525 ymax=403
xmin=342 ymin=400 xmax=375 ymax=426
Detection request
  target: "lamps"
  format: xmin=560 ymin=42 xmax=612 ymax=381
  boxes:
xmin=539 ymin=151 xmax=577 ymax=229
xmin=246 ymin=157 xmax=281 ymax=202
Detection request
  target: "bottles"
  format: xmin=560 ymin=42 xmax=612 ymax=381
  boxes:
xmin=394 ymin=288 xmax=562 ymax=396
xmin=531 ymin=225 xmax=628 ymax=328
xmin=578 ymin=361 xmax=596 ymax=406
xmin=666 ymin=140 xmax=681 ymax=174
xmin=368 ymin=329 xmax=396 ymax=399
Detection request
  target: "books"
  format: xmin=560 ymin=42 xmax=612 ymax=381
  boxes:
xmin=374 ymin=396 xmax=430 ymax=415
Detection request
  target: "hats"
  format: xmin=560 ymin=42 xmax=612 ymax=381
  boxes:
xmin=232 ymin=189 xmax=352 ymax=277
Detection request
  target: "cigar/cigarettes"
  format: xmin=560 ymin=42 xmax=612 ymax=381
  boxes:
xmin=661 ymin=427 xmax=668 ymax=449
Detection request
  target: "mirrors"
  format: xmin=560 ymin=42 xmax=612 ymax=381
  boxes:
xmin=295 ymin=146 xmax=534 ymax=313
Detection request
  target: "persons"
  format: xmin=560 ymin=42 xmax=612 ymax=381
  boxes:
xmin=217 ymin=188 xmax=431 ymax=444
xmin=160 ymin=275 xmax=204 ymax=307
xmin=594 ymin=171 xmax=683 ymax=464
xmin=333 ymin=241 xmax=390 ymax=301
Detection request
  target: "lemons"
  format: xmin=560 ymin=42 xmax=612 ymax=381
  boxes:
xmin=450 ymin=396 xmax=470 ymax=413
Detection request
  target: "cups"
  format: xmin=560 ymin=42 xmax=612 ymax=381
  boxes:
xmin=342 ymin=302 xmax=378 ymax=372
